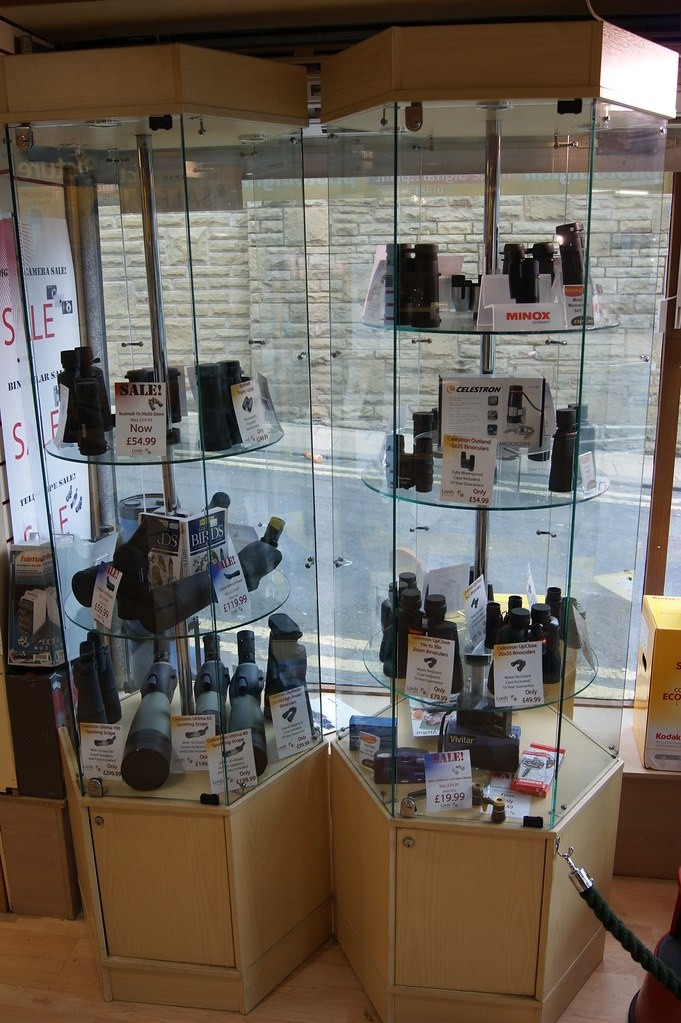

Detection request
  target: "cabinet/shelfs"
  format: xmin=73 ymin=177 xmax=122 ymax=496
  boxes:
xmin=319 ymin=19 xmax=681 ymax=1021
xmin=1 ymin=40 xmax=337 ymax=1013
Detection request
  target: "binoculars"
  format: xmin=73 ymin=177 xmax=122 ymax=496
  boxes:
xmin=487 ymin=604 xmax=563 ymax=695
xmin=386 ymin=434 xmax=433 ymax=493
xmin=196 ymin=361 xmax=252 ymax=451
xmin=503 ymin=222 xmax=593 ymax=304
xmin=413 ymin=408 xmax=438 ymax=438
xmin=74 ymin=630 xmax=122 ymax=734
xmin=485 ymin=596 xmax=522 ymax=649
xmin=547 ymin=403 xmax=596 ymax=492
xmin=546 ymin=587 xmax=586 ymax=649
xmin=382 ymin=589 xmax=465 ymax=695
xmin=379 ymin=572 xmax=419 ymax=662
xmin=385 ymin=243 xmax=441 ymax=328
xmin=58 ymin=346 xmax=113 ymax=444
xmin=264 ymin=613 xmax=315 ymax=738
